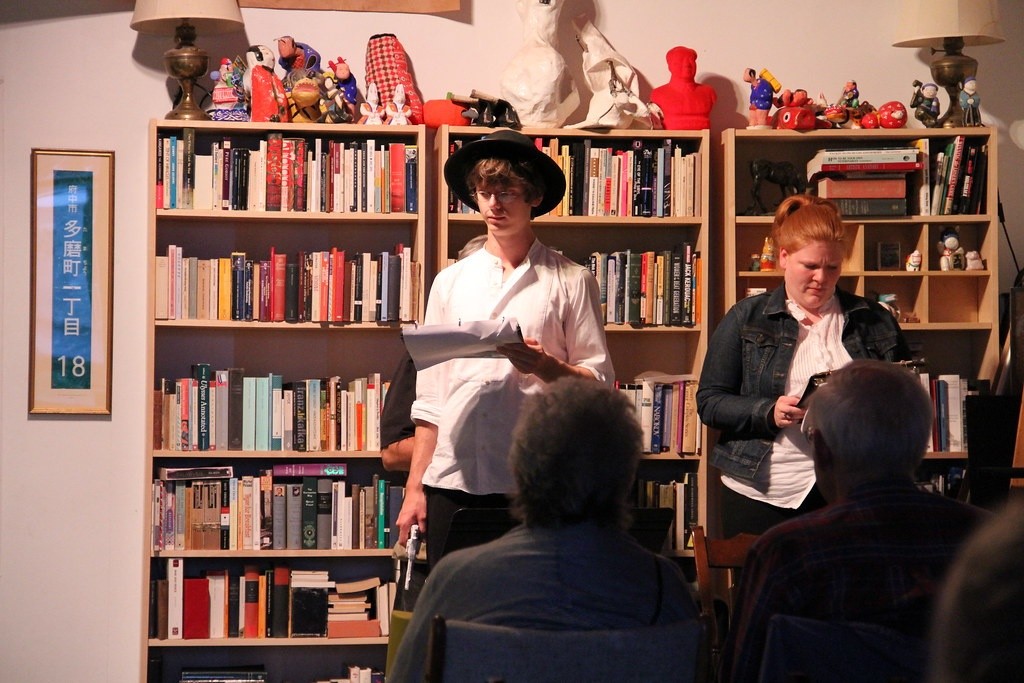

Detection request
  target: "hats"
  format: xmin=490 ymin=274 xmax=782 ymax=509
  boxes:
xmin=444 ymin=130 xmax=566 ymax=217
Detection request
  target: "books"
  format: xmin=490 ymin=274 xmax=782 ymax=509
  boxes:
xmin=154 ymin=359 xmax=411 ymax=549
xmin=614 ymin=376 xmax=702 ymax=552
xmin=147 ymin=556 xmax=397 ymax=683
xmin=157 ymin=125 xmax=420 ymax=324
xmin=447 ymin=136 xmax=703 ymax=328
xmin=805 ymin=133 xmax=988 ymax=218
xmin=915 ymin=371 xmax=990 ymax=506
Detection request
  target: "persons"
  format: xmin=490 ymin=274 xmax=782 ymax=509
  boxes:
xmin=694 ymin=195 xmax=914 ymax=585
xmin=315 ymin=72 xmax=356 ymax=124
xmin=917 ymin=83 xmax=940 ymax=116
xmin=274 ymin=34 xmax=321 ymax=90
xmin=501 ymin=0 xmax=567 ymax=129
xmin=958 ymin=76 xmax=982 ymax=127
xmin=387 ymin=375 xmax=703 ymax=683
xmin=245 ymin=45 xmax=293 ymax=121
xmin=772 ymin=88 xmax=814 ymax=107
xmin=839 ymin=80 xmax=860 ymax=107
xmin=741 ymin=68 xmax=773 ymax=127
xmin=650 ymin=46 xmax=716 ymax=118
xmin=930 ymin=498 xmax=1024 ymax=683
xmin=391 ymin=130 xmax=617 ymax=568
xmin=725 ymin=358 xmax=1007 ymax=683
xmin=335 ymin=62 xmax=357 ymax=121
xmin=211 ymin=58 xmax=238 ymax=109
xmin=379 ymin=234 xmax=488 ymax=612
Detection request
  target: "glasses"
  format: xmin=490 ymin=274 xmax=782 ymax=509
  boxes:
xmin=472 ymin=189 xmax=527 ymax=202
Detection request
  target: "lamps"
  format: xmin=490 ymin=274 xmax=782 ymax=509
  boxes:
xmin=891 ymin=0 xmax=1005 ymax=128
xmin=130 ymin=0 xmax=244 ymax=120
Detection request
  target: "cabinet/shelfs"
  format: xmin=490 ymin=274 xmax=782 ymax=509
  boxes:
xmin=433 ymin=124 xmax=710 ymax=557
xmin=140 ymin=120 xmax=425 ymax=683
xmin=716 ymin=128 xmax=999 ymax=506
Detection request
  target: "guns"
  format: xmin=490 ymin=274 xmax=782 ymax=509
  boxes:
xmin=404 ymin=524 xmax=422 ymax=590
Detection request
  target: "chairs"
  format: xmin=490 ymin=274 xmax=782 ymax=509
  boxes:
xmin=690 ymin=526 xmax=761 ymax=663
xmin=423 ymin=613 xmax=713 ymax=683
